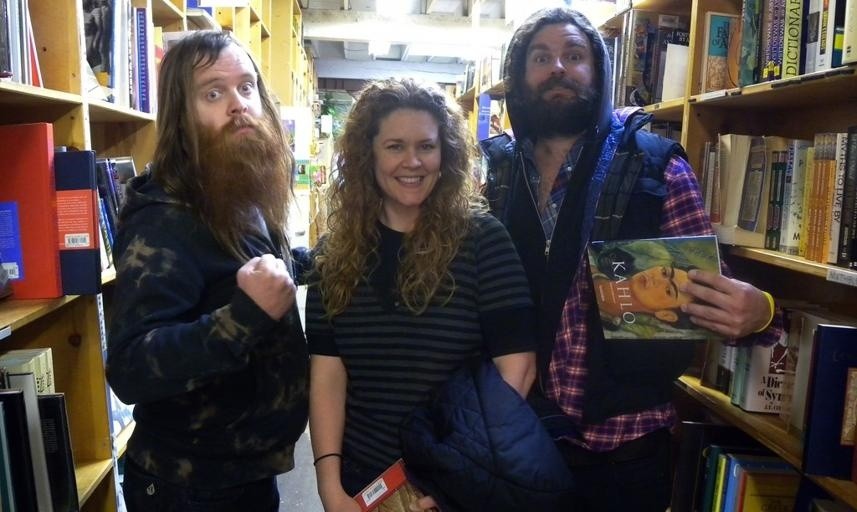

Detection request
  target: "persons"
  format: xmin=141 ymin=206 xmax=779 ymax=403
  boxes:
xmin=480 ymin=5 xmax=783 ymax=512
xmin=306 ymin=75 xmax=538 ymax=512
xmin=104 ymin=33 xmax=323 ymax=512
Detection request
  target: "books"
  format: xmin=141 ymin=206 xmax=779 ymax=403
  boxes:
xmin=354 ymin=458 xmax=437 ymax=512
xmin=453 ymin=42 xmax=511 ymax=194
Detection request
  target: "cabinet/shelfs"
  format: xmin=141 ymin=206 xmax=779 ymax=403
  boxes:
xmin=598 ymin=2 xmax=694 ymax=114
xmin=190 ymin=3 xmax=318 ymax=222
xmin=671 ymin=1 xmax=854 ymax=505
xmin=3 ymin=2 xmax=184 ymax=509
xmin=451 ymin=40 xmax=513 ymax=142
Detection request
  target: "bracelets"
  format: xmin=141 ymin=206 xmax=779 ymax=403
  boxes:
xmin=315 ymin=453 xmax=343 ymax=466
